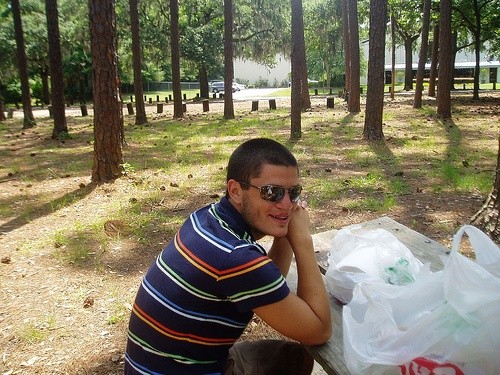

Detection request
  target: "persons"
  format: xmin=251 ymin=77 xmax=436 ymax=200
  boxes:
xmin=124 ymin=138 xmax=333 ymax=375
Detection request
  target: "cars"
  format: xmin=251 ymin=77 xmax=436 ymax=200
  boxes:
xmin=212 ymin=81 xmax=246 ymax=93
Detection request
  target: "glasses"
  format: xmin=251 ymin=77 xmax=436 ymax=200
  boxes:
xmin=239 ymin=181 xmax=304 ymax=202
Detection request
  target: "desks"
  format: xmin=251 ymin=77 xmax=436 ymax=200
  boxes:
xmin=263 ymin=216 xmax=474 ymax=375
